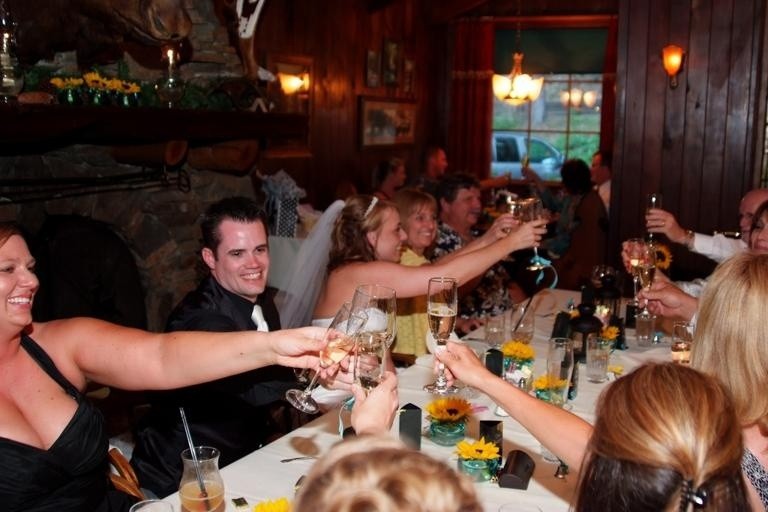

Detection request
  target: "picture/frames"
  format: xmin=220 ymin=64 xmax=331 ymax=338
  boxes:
xmin=363 ymin=35 xmax=416 ymax=100
xmin=355 ymin=95 xmax=420 ymax=150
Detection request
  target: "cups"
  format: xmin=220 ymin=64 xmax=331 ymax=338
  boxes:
xmin=586 ymin=336 xmax=609 ymax=383
xmin=634 ymin=316 xmax=656 ymax=347
xmin=283 ymin=301 xmax=368 ymax=417
xmin=179 ymin=447 xmax=224 ymax=512
xmin=669 ymin=320 xmax=696 ymax=369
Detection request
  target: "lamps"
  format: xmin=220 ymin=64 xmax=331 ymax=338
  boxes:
xmin=559 ymin=82 xmax=598 ymax=112
xmin=491 ymin=6 xmax=544 ymax=108
xmin=658 ymin=43 xmax=686 ymax=89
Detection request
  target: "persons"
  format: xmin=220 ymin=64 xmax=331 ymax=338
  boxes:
xmin=270 ymin=144 xmax=768 ymax=421
xmin=127 ymin=197 xmax=380 ymax=499
xmin=433 ymin=339 xmax=751 ymax=512
xmin=688 ymin=254 xmax=767 ymax=512
xmin=294 ymin=371 xmax=479 ymax=512
xmin=0 ymin=224 xmax=363 ymax=512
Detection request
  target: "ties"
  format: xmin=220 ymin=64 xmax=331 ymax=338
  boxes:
xmin=251 ymin=304 xmax=269 ymax=332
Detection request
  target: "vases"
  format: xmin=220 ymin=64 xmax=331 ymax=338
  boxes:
xmin=94 ymin=89 xmax=100 ymax=106
xmin=67 ymin=87 xmax=73 ymax=103
xmin=123 ymin=93 xmax=130 ymax=106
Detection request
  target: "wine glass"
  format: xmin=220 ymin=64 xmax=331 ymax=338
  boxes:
xmin=632 ymin=244 xmax=658 ymax=322
xmin=510 ymin=304 xmax=537 ymax=345
xmin=515 ymin=197 xmax=552 ymax=272
xmin=426 ymin=276 xmax=458 ymax=396
xmin=348 ymin=284 xmax=398 ymax=394
xmin=642 ymin=192 xmax=663 ymax=246
xmin=483 ymin=309 xmax=505 ymax=350
xmin=545 ymin=335 xmax=571 ymax=413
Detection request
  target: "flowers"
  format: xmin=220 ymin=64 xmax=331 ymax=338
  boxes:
xmin=109 ymin=79 xmax=142 ymax=100
xmin=84 ymin=70 xmax=108 ymax=92
xmin=49 ymin=72 xmax=81 ymax=88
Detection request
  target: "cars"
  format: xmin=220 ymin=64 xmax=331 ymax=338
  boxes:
xmin=491 ymin=132 xmax=568 ymax=184
